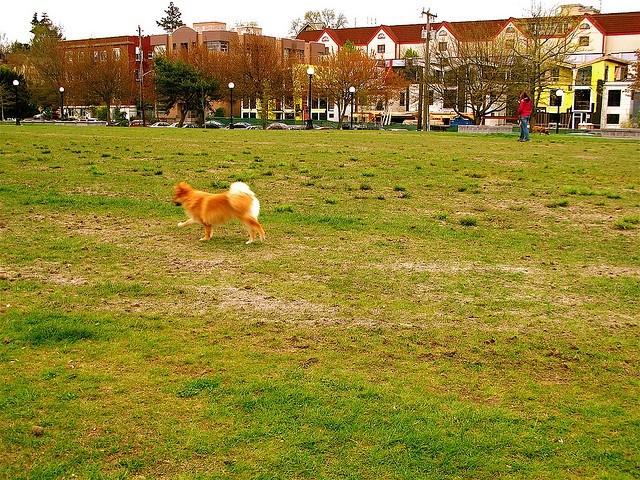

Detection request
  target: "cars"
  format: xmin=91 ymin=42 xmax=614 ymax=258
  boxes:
xmin=150 ymin=122 xmax=170 ymax=127
xmin=170 ymin=123 xmax=186 ymax=128
xmin=130 ymin=121 xmax=144 ymax=126
xmin=227 ymin=124 xmax=247 ymax=130
xmin=202 ymin=123 xmax=221 ymax=128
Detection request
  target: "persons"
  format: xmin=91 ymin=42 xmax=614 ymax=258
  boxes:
xmin=516 ymin=91 xmax=533 ymax=142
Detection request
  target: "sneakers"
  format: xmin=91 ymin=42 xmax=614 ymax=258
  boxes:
xmin=520 ymin=139 xmax=530 ymax=142
xmin=517 ymin=138 xmax=523 ymax=141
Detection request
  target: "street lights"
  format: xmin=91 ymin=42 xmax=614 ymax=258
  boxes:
xmin=349 ymin=86 xmax=355 ymax=130
xmin=556 ymin=90 xmax=563 ymax=134
xmin=13 ymin=80 xmax=20 ymax=125
xmin=228 ymin=82 xmax=235 ymax=129
xmin=306 ymin=68 xmax=314 ymax=129
xmin=59 ymin=87 xmax=64 ymax=121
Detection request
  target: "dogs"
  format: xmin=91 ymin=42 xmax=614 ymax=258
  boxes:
xmin=531 ymin=125 xmax=549 ymax=134
xmin=171 ymin=182 xmax=266 ymax=244
